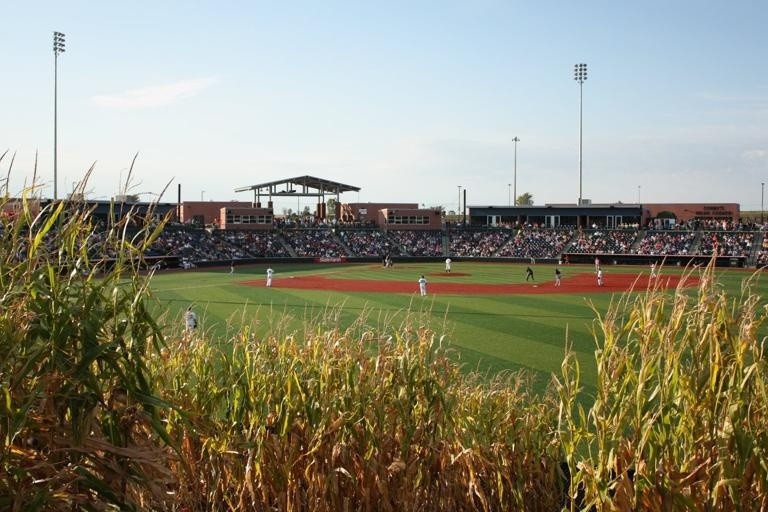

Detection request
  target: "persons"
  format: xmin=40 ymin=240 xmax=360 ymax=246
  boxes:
xmin=266 ymin=265 xmax=274 ymax=287
xmin=597 ymin=268 xmax=602 ymax=287
xmin=457 ymin=230 xmax=510 ymax=258
xmin=495 ymin=218 xmax=768 ymax=266
xmin=526 ymin=267 xmax=534 ymax=282
xmin=554 ymin=269 xmax=561 ymax=288
xmin=650 ymin=262 xmax=657 ymax=279
xmin=446 ymin=257 xmax=451 ymax=273
xmin=6 ymin=218 xmax=293 ymax=267
xmin=230 ymin=261 xmax=234 ymax=273
xmin=418 ymin=275 xmax=428 ymax=297
xmin=290 ymin=218 xmax=467 ymax=266
xmin=184 ymin=306 xmax=197 ymax=333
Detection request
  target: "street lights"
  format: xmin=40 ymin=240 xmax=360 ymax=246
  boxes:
xmin=507 ymin=184 xmax=512 ymax=206
xmin=638 ymin=185 xmax=641 ymax=204
xmin=574 ymin=63 xmax=588 ymax=199
xmin=457 ymin=185 xmax=462 ymax=219
xmin=512 ymin=135 xmax=520 ymax=204
xmin=53 ymin=31 xmax=65 ymax=200
xmin=761 ymin=182 xmax=764 ymax=224
